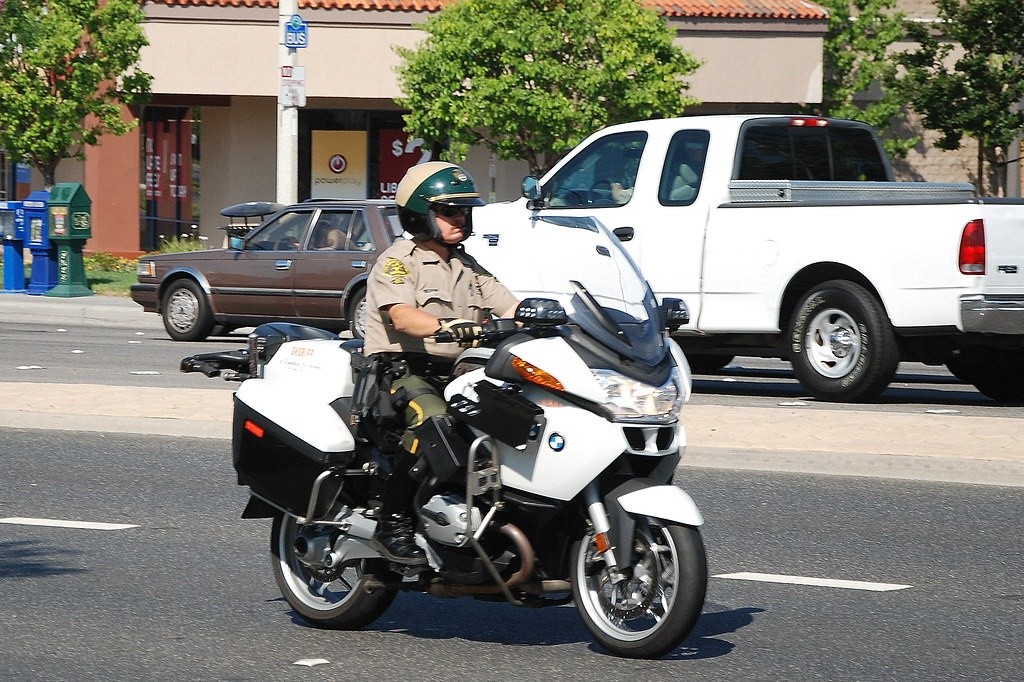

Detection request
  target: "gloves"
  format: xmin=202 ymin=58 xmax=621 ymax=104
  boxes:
xmin=434 ymin=315 xmax=484 ymax=346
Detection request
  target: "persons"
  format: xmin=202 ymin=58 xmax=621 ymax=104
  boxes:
xmin=611 ymin=183 xmax=634 ymax=204
xmin=354 ymin=161 xmax=522 ymax=566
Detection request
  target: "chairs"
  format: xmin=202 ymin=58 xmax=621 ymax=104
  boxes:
xmin=312 ymin=227 xmax=342 ymax=251
xmin=666 ymin=160 xmax=703 ymax=203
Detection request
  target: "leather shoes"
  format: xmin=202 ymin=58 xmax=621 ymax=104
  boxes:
xmin=368 ymin=512 xmax=428 ymax=566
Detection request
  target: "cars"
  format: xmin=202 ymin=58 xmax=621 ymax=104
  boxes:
xmin=130 ymin=195 xmax=403 ymax=340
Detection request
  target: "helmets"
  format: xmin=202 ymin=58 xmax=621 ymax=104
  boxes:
xmin=394 ymin=161 xmax=487 ymax=243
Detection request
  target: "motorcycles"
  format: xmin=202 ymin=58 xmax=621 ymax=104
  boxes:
xmin=181 ymin=214 xmax=710 ymax=664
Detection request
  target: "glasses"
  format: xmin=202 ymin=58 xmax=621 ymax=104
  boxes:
xmin=434 ymin=203 xmax=469 ymax=218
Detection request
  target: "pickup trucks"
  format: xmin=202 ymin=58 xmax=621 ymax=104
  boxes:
xmin=389 ymin=110 xmax=1024 ymax=405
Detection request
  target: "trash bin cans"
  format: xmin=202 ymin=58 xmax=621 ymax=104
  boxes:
xmin=218 ymin=200 xmax=285 ymax=251
